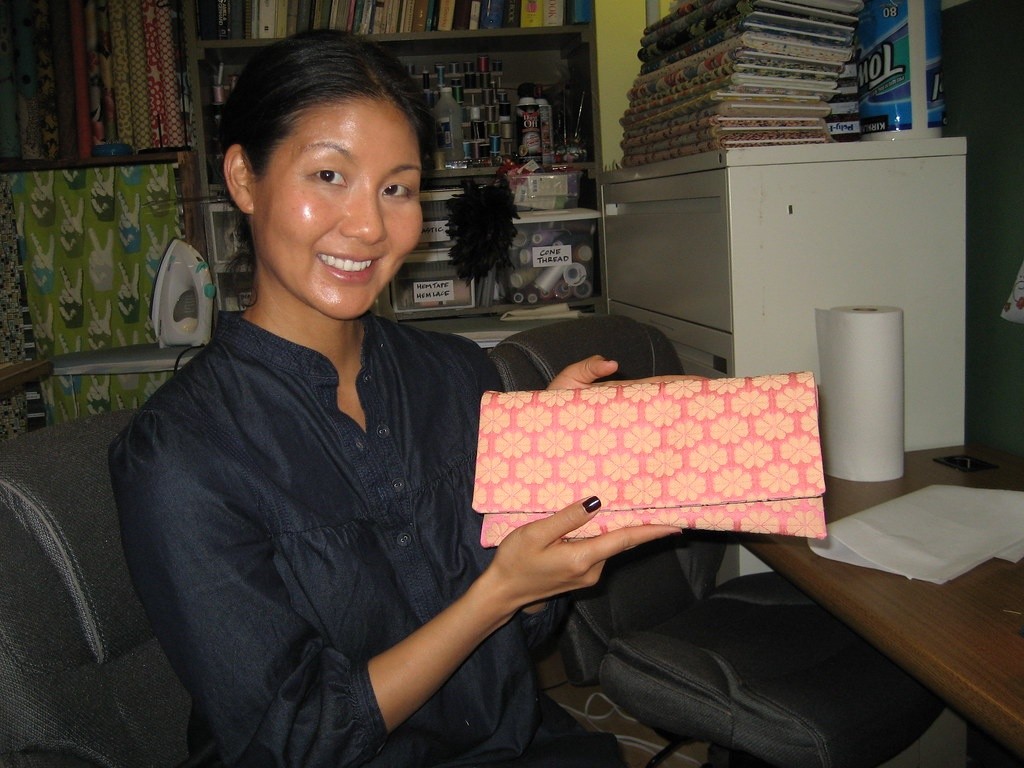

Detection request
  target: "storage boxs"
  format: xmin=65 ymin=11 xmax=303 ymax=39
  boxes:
xmin=392 ymin=189 xmax=476 ymax=313
xmin=505 ymin=206 xmax=601 ymax=308
xmin=204 ymin=202 xmax=253 ymax=313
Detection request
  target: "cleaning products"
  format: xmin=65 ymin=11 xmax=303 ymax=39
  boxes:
xmin=432 ymin=87 xmax=465 ymax=161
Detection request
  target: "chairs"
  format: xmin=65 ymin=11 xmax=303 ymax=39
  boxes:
xmin=489 ymin=315 xmax=942 ymax=768
xmin=0 ymin=410 xmax=195 ymax=768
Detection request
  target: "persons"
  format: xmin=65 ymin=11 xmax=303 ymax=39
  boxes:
xmin=108 ymin=28 xmax=710 ymax=768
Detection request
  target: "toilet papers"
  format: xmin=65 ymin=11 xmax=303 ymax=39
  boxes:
xmin=856 ymin=0 xmax=942 ymax=142
xmin=815 ymin=304 xmax=905 ymax=482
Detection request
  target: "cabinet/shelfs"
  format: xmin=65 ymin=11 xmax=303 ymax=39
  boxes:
xmin=0 ymin=0 xmax=207 ymax=440
xmin=598 ymin=137 xmax=967 ymax=586
xmin=181 ymin=0 xmax=604 ymax=363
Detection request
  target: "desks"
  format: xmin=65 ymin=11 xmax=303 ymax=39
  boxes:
xmin=741 ymin=444 xmax=1024 ymax=757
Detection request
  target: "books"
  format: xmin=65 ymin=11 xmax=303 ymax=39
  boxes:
xmin=198 ymin=0 xmax=593 ymax=40
